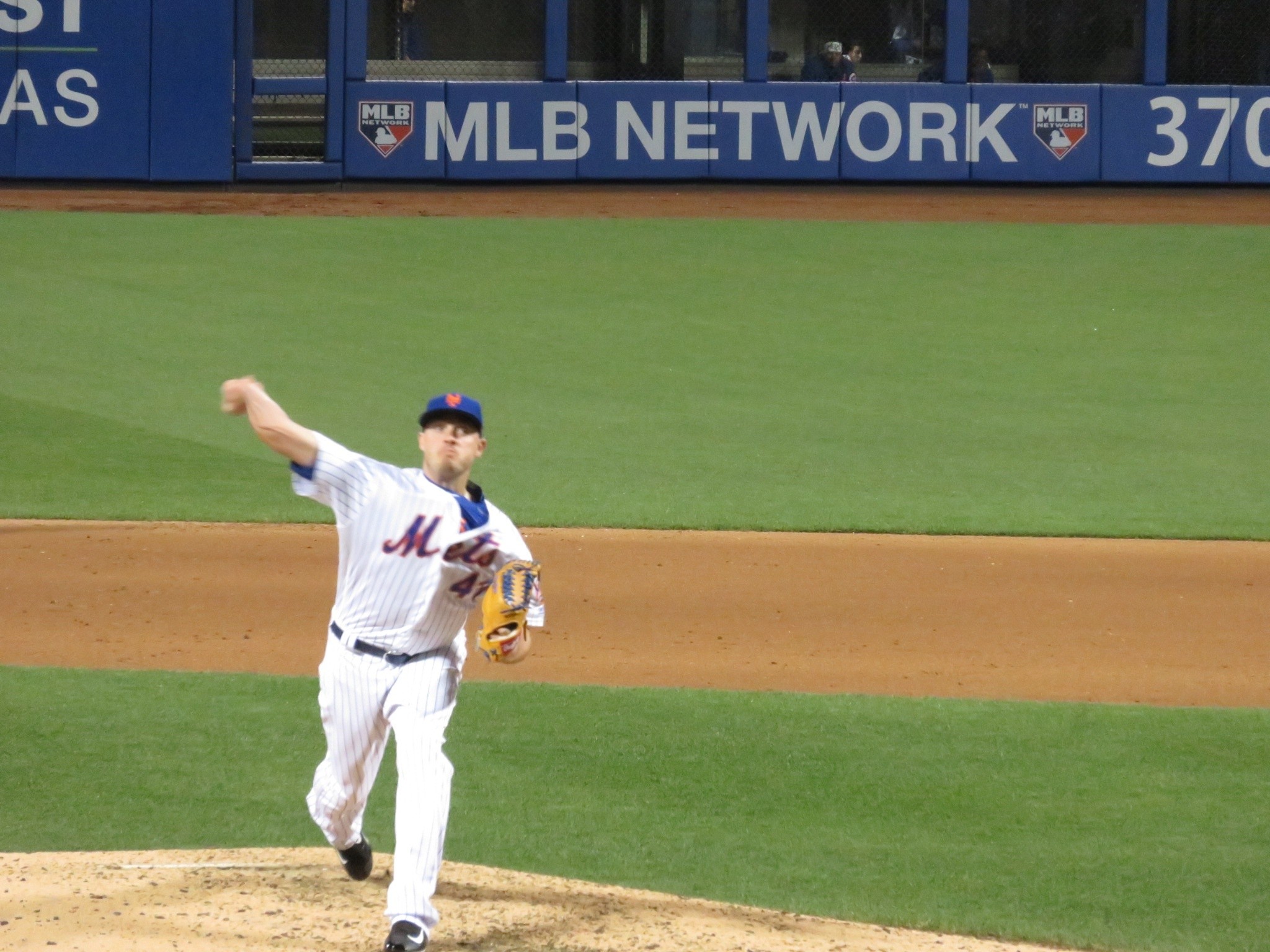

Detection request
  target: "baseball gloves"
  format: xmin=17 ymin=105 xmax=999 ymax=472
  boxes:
xmin=475 ymin=559 xmax=542 ymax=664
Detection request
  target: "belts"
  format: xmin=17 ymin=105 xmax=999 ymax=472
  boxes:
xmin=328 ymin=621 xmax=419 ymax=665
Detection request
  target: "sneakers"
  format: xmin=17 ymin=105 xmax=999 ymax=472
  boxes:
xmin=383 ymin=920 xmax=428 ymax=952
xmin=336 ymin=832 xmax=373 ymax=881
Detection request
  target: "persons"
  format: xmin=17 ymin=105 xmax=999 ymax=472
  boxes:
xmin=801 ymin=0 xmax=996 ymax=83
xmin=219 ymin=375 xmax=544 ymax=952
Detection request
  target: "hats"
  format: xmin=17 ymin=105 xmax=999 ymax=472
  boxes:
xmin=821 ymin=41 xmax=842 ymax=53
xmin=419 ymin=393 xmax=482 ymax=429
